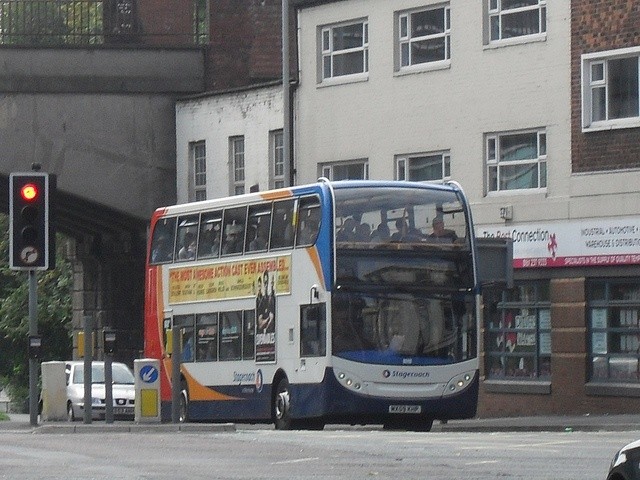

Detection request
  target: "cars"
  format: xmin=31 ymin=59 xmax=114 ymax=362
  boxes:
xmin=65 ymin=360 xmax=134 ymax=422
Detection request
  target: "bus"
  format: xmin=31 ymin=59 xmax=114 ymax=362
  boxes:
xmin=145 ymin=178 xmax=482 ymax=432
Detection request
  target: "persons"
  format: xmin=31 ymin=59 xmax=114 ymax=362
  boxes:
xmin=391 ymin=219 xmax=423 ymax=243
xmin=427 ymin=216 xmax=460 ymax=244
xmin=255 ymin=276 xmax=263 ymax=333
xmin=372 ymin=223 xmax=392 ymax=243
xmin=150 ymin=235 xmax=172 ymax=263
xmin=256 ymin=269 xmax=276 ymax=334
xmin=268 ymin=273 xmax=277 ymax=332
xmin=174 ymin=207 xmax=320 ymax=260
xmin=355 ymin=223 xmax=372 ymax=244
xmin=335 ymin=217 xmax=357 ymax=243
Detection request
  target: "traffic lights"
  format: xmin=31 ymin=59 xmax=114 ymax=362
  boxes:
xmin=10 ymin=171 xmax=47 ymax=270
